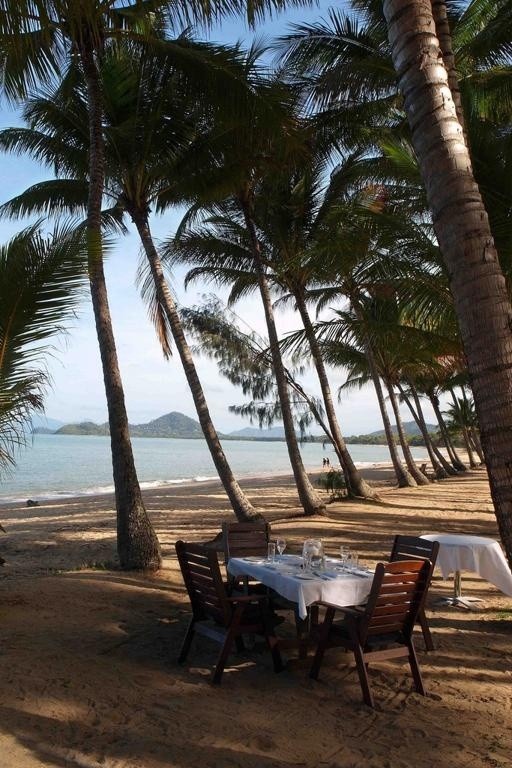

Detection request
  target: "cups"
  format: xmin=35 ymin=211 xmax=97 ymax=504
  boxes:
xmin=304 ymin=551 xmax=311 ymax=575
xmin=268 ymin=543 xmax=276 ymax=563
xmin=351 ymin=551 xmax=358 ymax=569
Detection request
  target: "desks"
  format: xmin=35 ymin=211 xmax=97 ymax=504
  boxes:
xmin=418 ymin=534 xmax=512 ymax=612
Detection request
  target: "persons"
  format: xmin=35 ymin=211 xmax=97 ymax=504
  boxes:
xmin=327 ymin=458 xmax=330 ymax=466
xmin=322 ymin=458 xmax=326 ymax=467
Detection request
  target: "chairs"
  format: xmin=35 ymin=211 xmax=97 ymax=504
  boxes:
xmin=222 ymin=521 xmax=271 ymax=596
xmin=309 ymin=558 xmax=434 ymax=709
xmin=389 ymin=535 xmax=440 ymax=650
xmin=174 ymin=541 xmax=285 ymax=688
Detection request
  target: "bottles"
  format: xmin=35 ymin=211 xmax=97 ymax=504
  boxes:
xmin=302 ymin=539 xmax=324 ymax=573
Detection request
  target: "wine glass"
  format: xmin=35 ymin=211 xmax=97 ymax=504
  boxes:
xmin=341 ymin=545 xmax=349 ymax=570
xmin=277 ymin=538 xmax=286 ymax=565
